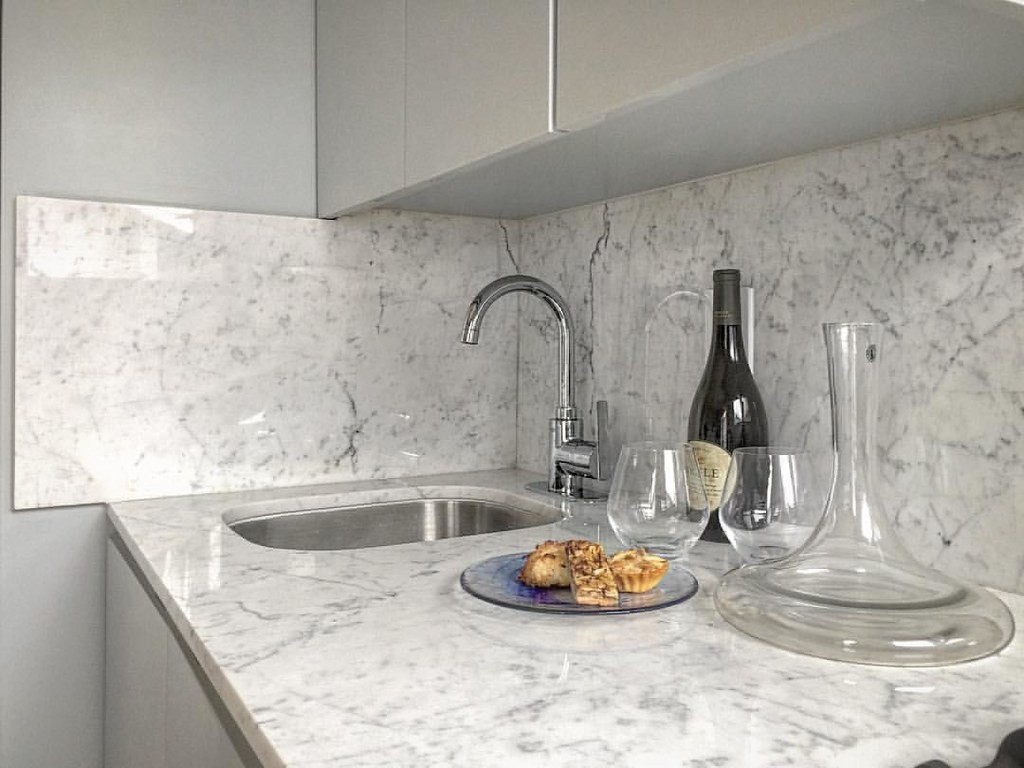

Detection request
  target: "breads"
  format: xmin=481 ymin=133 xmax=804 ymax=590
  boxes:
xmin=606 ymin=548 xmax=668 ymax=593
xmin=565 ymin=540 xmax=619 ymax=606
xmin=513 ymin=538 xmax=572 ymax=588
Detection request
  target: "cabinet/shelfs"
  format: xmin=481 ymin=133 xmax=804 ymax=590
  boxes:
xmin=316 ymin=0 xmax=1024 ymax=220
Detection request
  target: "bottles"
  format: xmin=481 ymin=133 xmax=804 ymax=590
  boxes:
xmin=713 ymin=318 xmax=1016 ymax=668
xmin=684 ymin=269 xmax=770 ymax=542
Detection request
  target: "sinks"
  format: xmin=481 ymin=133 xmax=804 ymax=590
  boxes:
xmin=219 ymin=480 xmax=576 ymax=554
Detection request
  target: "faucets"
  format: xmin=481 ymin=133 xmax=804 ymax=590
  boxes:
xmin=459 ymin=273 xmax=586 ymax=494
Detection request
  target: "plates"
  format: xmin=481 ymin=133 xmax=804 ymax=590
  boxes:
xmin=462 ymin=550 xmax=698 ymax=617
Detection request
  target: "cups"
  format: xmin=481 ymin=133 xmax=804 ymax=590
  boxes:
xmin=608 ymin=441 xmax=712 ymax=561
xmin=717 ymin=445 xmax=825 ymax=565
